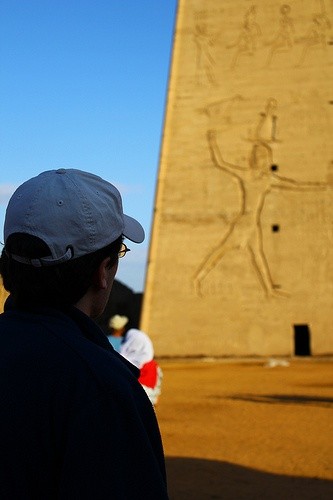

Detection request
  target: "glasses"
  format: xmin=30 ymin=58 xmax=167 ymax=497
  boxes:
xmin=109 ymin=243 xmax=130 ymax=259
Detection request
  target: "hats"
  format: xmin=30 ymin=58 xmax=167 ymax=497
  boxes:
xmin=0 ymin=168 xmax=147 ymax=266
xmin=108 ymin=314 xmax=129 ymax=329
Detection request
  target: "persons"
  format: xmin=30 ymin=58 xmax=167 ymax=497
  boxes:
xmin=104 ymin=313 xmax=129 ymax=351
xmin=1 ymin=167 xmax=170 ymax=500
